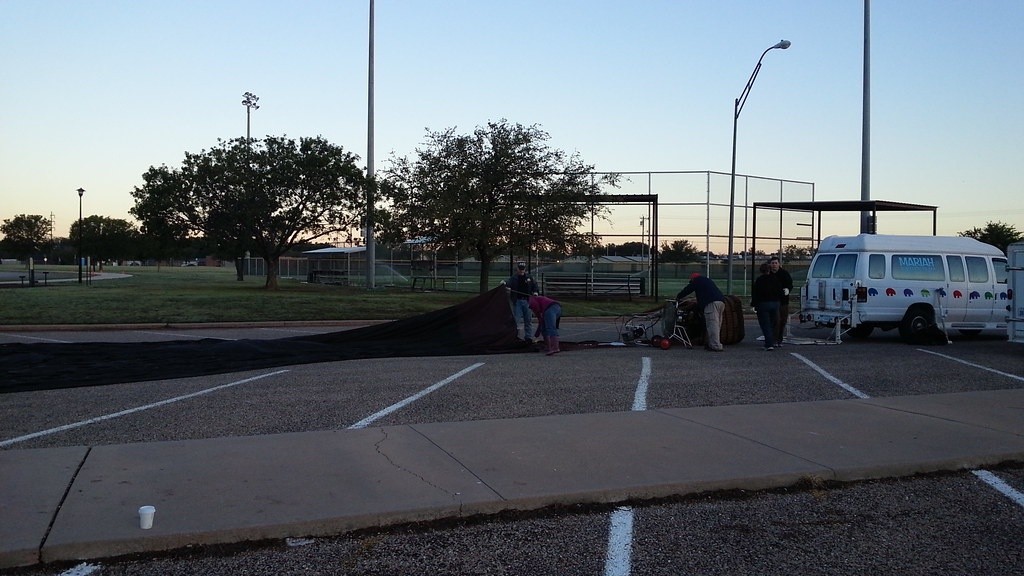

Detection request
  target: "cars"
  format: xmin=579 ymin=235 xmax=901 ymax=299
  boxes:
xmin=128 ymin=260 xmax=144 ymax=266
xmin=181 ymin=260 xmax=205 ymax=266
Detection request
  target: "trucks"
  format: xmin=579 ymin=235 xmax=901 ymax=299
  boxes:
xmin=1002 ymin=242 xmax=1024 ymax=347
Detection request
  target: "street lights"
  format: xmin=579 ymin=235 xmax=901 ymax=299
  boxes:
xmin=727 ymin=40 xmax=793 ymax=297
xmin=243 ymin=91 xmax=259 ymax=151
xmin=78 ymin=188 xmax=84 ymax=283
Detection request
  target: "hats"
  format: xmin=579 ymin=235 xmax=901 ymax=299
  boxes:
xmin=517 ymin=261 xmax=526 ymax=270
xmin=688 ymin=272 xmax=700 ymax=283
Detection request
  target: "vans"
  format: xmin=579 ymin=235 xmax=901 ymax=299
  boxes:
xmin=800 ymin=235 xmax=1008 ymax=343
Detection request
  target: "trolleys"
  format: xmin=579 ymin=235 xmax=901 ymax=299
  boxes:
xmin=651 ymin=299 xmax=692 ymax=350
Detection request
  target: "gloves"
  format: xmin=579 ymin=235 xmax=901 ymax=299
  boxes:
xmin=752 ymin=307 xmax=757 ymax=313
xmin=500 ymin=280 xmax=507 ymax=285
xmin=783 ymin=288 xmax=790 ymax=296
xmin=533 ymin=292 xmax=539 ymax=296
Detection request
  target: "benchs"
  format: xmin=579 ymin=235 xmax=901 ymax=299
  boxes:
xmin=542 ymin=272 xmax=645 ymax=299
xmin=409 ymin=276 xmax=455 ymax=289
xmin=313 ymin=269 xmax=351 ymax=285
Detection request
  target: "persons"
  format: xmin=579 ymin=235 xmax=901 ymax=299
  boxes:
xmin=676 ymin=273 xmax=726 ymax=352
xmin=500 ymin=262 xmax=562 ymax=356
xmin=751 ymin=257 xmax=793 ymax=351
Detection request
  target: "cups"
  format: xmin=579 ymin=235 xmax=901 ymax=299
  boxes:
xmin=138 ymin=505 xmax=156 ymax=529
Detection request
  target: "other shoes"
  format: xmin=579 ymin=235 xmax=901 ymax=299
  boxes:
xmin=779 ymin=343 xmax=783 ymax=347
xmin=774 ymin=342 xmax=778 ymax=347
xmin=768 ymin=347 xmax=774 ymax=349
xmin=706 ymin=345 xmax=724 ymax=352
xmin=525 ymin=337 xmax=536 ymax=342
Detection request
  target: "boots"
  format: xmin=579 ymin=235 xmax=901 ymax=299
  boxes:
xmin=552 ymin=335 xmax=561 ymax=352
xmin=543 ymin=335 xmax=555 ymax=356
